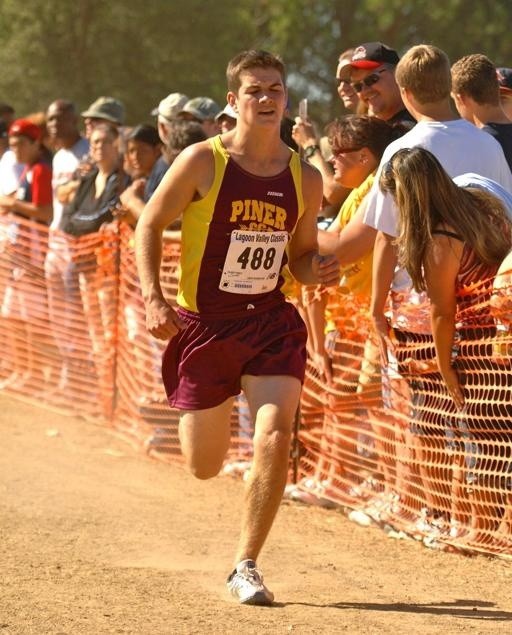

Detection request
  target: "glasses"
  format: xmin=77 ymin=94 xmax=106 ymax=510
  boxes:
xmin=329 ymin=146 xmax=363 ymax=154
xmin=336 ymin=76 xmax=351 ymax=87
xmin=350 ymin=69 xmax=387 ymax=92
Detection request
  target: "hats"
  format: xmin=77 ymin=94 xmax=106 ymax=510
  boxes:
xmin=10 ymin=119 xmax=42 ymax=141
xmin=495 ymin=67 xmax=512 ymax=98
xmin=336 ymin=47 xmax=356 ymax=79
xmin=177 ymin=97 xmax=220 ymax=121
xmin=214 ymin=102 xmax=239 ymax=120
xmin=81 ymin=97 xmax=125 ymax=124
xmin=151 ymin=92 xmax=186 ymax=120
xmin=350 ymin=42 xmax=400 ymax=70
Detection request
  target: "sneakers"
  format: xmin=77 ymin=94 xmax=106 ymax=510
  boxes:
xmin=228 ymin=559 xmax=274 ymax=604
xmin=5 ymin=371 xmax=79 ymax=408
xmin=222 ymin=460 xmax=466 ymax=549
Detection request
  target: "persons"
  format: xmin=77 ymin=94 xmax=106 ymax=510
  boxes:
xmin=279 ymin=42 xmax=511 ymax=557
xmin=1 ymin=93 xmax=254 ymax=480
xmin=134 ymin=46 xmax=346 ymax=606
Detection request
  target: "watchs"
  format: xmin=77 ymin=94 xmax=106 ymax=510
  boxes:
xmin=304 ymin=145 xmax=319 ymax=162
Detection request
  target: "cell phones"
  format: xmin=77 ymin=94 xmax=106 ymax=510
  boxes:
xmin=296 ymin=97 xmax=309 ymax=122
